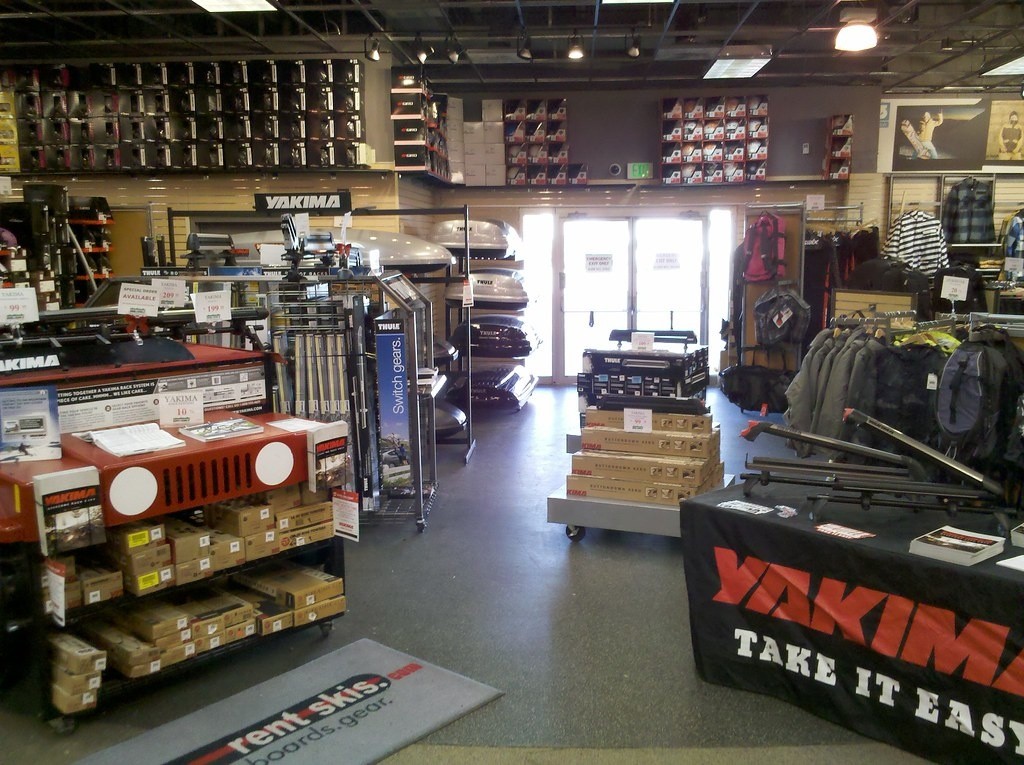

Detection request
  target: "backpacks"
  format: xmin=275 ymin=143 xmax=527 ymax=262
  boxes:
xmin=753 ymin=287 xmax=790 ymax=345
xmin=935 ymin=325 xmax=1024 ymax=474
xmin=743 ymin=209 xmax=786 ymax=283
xmin=785 ymin=287 xmax=810 ymax=344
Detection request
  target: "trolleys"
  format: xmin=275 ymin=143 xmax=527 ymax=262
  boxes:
xmin=542 ymin=327 xmax=735 ymax=541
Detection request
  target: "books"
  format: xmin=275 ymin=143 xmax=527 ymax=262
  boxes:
xmin=307 ymin=419 xmax=350 ymax=492
xmin=177 ymin=417 xmax=265 ymax=444
xmin=70 ymin=422 xmax=187 ymax=457
xmin=908 ymin=524 xmax=1006 ymax=567
xmin=1010 ymin=522 xmax=1024 ymax=548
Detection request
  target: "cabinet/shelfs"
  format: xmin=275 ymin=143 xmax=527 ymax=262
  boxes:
xmin=68 ymin=219 xmax=117 ymax=307
xmin=823 ymin=114 xmax=854 ymax=181
xmin=446 ymin=256 xmax=533 ymax=411
xmin=417 ymin=124 xmax=449 ymax=185
xmin=658 ymin=94 xmax=771 ymax=185
xmin=502 ymin=97 xmax=587 ymax=185
xmin=81 ymin=271 xmax=438 ymax=533
xmin=167 ymin=204 xmax=477 ymax=464
xmin=390 ymin=88 xmax=427 ymax=170
xmin=883 ymin=171 xmax=1003 ymax=271
xmin=0 ymin=536 xmax=345 ymax=734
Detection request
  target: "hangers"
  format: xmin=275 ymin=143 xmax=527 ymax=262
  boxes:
xmin=901 ymin=320 xmax=941 ymax=346
xmin=808 ymin=207 xmax=879 ymax=240
xmin=885 ymin=310 xmax=918 ymax=330
xmin=929 ymin=314 xmax=989 ymax=333
xmin=830 ymin=316 xmax=886 ymax=341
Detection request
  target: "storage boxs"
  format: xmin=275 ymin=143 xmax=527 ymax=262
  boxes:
xmin=391 ymin=67 xmax=449 ymax=177
xmin=42 ymin=484 xmax=335 ymax=615
xmin=566 ymin=342 xmax=724 ymax=506
xmin=447 ymin=97 xmax=506 ymax=187
xmin=0 ymin=58 xmax=367 ymax=173
xmin=44 ymin=560 xmax=348 ymax=714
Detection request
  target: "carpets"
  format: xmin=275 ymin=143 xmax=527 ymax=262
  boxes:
xmin=72 ymin=638 xmax=507 ymax=765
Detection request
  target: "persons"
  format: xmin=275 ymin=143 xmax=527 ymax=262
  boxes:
xmin=911 ymin=107 xmax=944 ymax=160
xmin=997 ymin=109 xmax=1023 ymax=159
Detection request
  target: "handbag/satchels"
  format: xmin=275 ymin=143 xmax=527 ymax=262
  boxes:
xmin=720 ymin=347 xmax=800 ymax=412
xmin=848 ymin=254 xmax=907 ymax=290
xmin=902 ymin=268 xmax=930 ymax=305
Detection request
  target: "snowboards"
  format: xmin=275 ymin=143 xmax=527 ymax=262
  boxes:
xmin=899 ymin=119 xmax=931 ymax=160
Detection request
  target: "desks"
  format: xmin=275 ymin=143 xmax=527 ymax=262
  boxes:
xmin=679 ymin=473 xmax=1024 ymax=765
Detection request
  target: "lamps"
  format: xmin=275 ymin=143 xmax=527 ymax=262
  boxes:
xmin=624 ymin=27 xmax=642 ymax=57
xmin=448 ymin=44 xmax=467 ymax=64
xmin=836 ymin=7 xmax=877 ymax=50
xmin=518 ymin=34 xmax=532 ymax=59
xmin=417 ymin=44 xmax=434 ymax=64
xmin=978 ymin=47 xmax=1024 ymax=75
xmin=366 ymin=39 xmax=380 ymax=61
xmin=566 ymin=28 xmax=586 ymax=58
xmin=702 ymin=45 xmax=772 ymax=79
